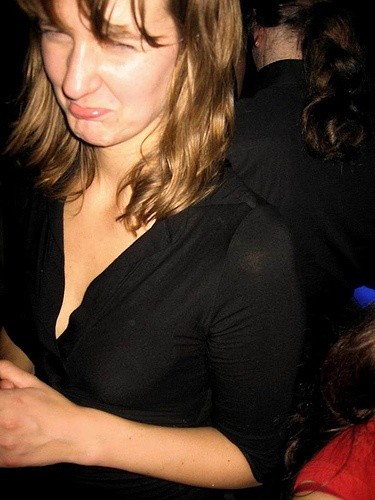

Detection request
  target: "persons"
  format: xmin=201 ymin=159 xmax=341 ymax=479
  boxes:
xmin=284 ymin=310 xmax=375 ymax=500
xmin=0 ymin=0 xmax=322 ymax=500
xmin=214 ymin=0 xmax=375 ymax=386
